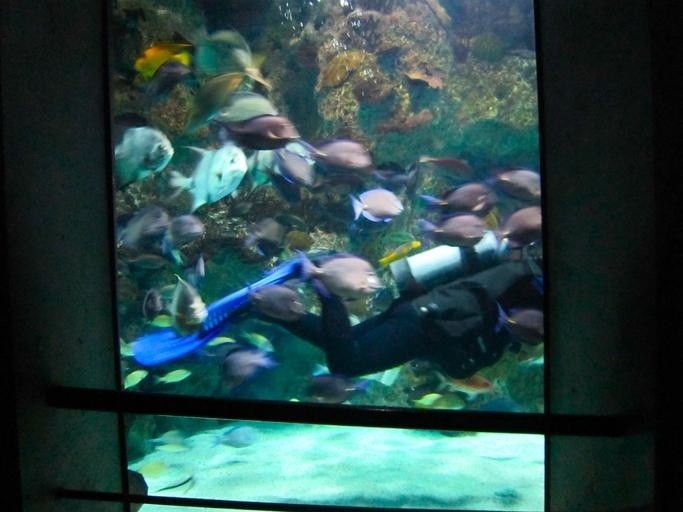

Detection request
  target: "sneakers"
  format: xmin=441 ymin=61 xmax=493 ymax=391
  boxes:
xmin=228 ymin=304 xmax=253 ymax=324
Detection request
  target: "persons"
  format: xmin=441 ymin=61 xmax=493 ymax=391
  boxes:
xmin=250 ymin=255 xmax=544 ymax=379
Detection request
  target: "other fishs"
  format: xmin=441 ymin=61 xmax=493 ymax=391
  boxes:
xmin=112 ymin=12 xmax=543 ymax=411
xmin=133 ymin=424 xmax=264 ymax=499
